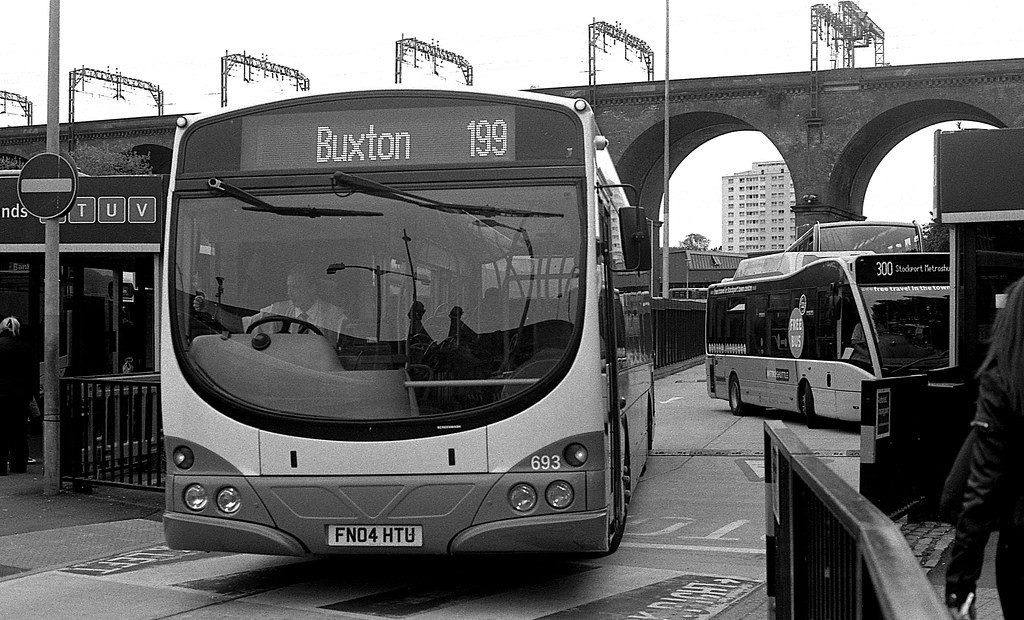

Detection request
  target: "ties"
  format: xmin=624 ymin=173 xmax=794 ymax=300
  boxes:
xmin=295 ymin=313 xmax=311 ymax=335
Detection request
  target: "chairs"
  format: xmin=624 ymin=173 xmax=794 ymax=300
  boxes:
xmin=423 ymin=303 xmax=460 ymax=339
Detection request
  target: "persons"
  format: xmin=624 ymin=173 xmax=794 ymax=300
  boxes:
xmin=945 ymin=275 xmax=1024 ymax=620
xmin=0 ymin=316 xmax=33 ymax=476
xmin=193 ymin=263 xmax=348 ymax=350
xmin=851 ymin=312 xmax=889 ymax=359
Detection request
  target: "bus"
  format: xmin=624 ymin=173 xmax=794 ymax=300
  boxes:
xmin=704 ymin=250 xmax=950 ymax=430
xmin=669 ymin=287 xmax=708 ymax=303
xmin=160 ymin=83 xmax=655 ymax=558
xmin=784 ymin=220 xmax=924 ymax=252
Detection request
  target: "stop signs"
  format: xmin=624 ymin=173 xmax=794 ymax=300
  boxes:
xmin=16 ymin=153 xmax=77 ymax=219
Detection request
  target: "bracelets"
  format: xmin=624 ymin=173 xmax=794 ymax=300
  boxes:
xmin=212 ymin=304 xmax=218 ymax=319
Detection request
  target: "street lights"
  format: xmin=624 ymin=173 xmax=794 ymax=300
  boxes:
xmin=471 ymin=219 xmax=535 ymax=370
xmin=326 ymin=262 xmax=430 ymax=341
